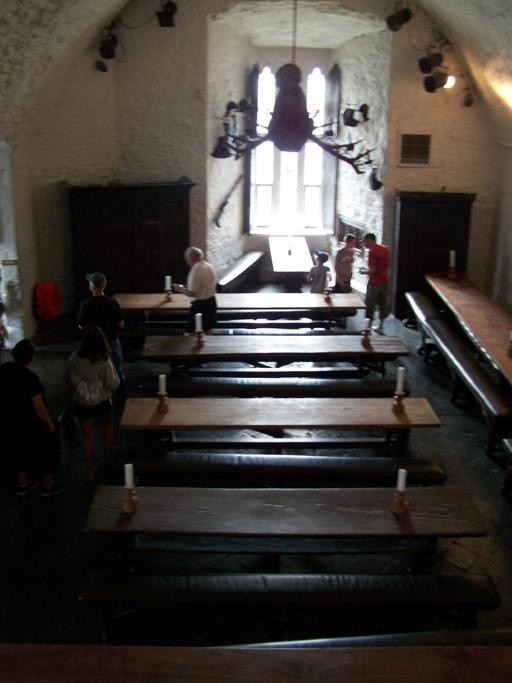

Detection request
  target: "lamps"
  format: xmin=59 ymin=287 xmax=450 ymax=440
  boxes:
xmin=211 ymin=0 xmax=376 ymax=174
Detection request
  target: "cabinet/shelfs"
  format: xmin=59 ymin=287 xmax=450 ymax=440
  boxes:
xmin=69 ymin=181 xmax=196 ymax=347
xmin=391 ymin=192 xmax=476 ymax=321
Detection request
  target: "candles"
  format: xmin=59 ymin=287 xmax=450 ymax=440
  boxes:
xmin=164 ymin=276 xmax=172 ymax=301
xmin=449 ymin=250 xmax=456 ymax=278
xmin=324 ymin=271 xmax=333 ymax=300
xmin=508 ymin=329 xmax=512 ymax=356
xmin=361 ymin=318 xmax=371 ymax=345
xmin=392 ymin=469 xmax=407 ymax=514
xmin=194 ymin=313 xmax=204 ymax=344
xmin=121 ymin=463 xmax=135 ymax=513
xmin=156 ymin=374 xmax=169 ymax=413
xmin=288 ymin=243 xmax=291 ymax=255
xmin=392 ymin=367 xmax=405 ymax=407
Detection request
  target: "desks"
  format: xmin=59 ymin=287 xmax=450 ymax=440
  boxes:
xmin=85 ymin=486 xmax=487 ymax=574
xmin=268 ymin=236 xmax=314 ymax=293
xmin=425 ymin=272 xmax=512 ymax=408
xmin=121 ymin=399 xmax=441 ymax=478
xmin=112 ymin=294 xmax=366 ymax=335
xmin=136 ymin=334 xmax=410 ymax=385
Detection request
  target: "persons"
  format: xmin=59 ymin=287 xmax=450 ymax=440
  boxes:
xmin=63 ymin=326 xmax=121 ymax=483
xmin=307 ymin=250 xmax=332 ymax=293
xmin=0 ymin=302 xmax=9 ymax=347
xmin=335 ymin=234 xmax=356 ymax=293
xmin=76 ymin=272 xmax=126 ymax=382
xmin=0 ymin=339 xmax=66 ymax=497
xmin=173 ymin=246 xmax=217 ymax=336
xmin=359 ymin=234 xmax=390 ymax=335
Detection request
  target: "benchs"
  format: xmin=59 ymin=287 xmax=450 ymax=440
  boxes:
xmin=142 ymin=451 xmax=446 ymax=486
xmin=503 ymin=439 xmax=512 ymax=492
xmin=313 ymin=252 xmax=338 ymax=292
xmin=64 ymin=573 xmax=502 ymax=644
xmin=217 ymin=250 xmax=266 ymax=294
xmin=404 ymin=291 xmax=512 ymax=457
xmin=176 ymin=377 xmax=410 ymax=399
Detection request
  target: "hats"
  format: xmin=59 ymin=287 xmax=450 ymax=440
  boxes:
xmin=359 ymin=233 xmax=376 ymax=242
xmin=86 ymin=272 xmax=107 ymax=288
xmin=314 ymin=249 xmax=330 ymax=263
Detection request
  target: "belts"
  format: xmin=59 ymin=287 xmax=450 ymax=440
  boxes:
xmin=194 ymin=299 xmax=214 ymax=303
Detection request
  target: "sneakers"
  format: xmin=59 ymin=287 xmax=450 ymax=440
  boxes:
xmin=104 ymin=448 xmax=118 ymax=465
xmin=83 ymin=465 xmax=97 ymax=485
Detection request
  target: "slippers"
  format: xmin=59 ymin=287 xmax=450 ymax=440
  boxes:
xmin=373 ymin=325 xmax=386 ymax=336
xmin=362 ymin=325 xmax=372 ymax=335
